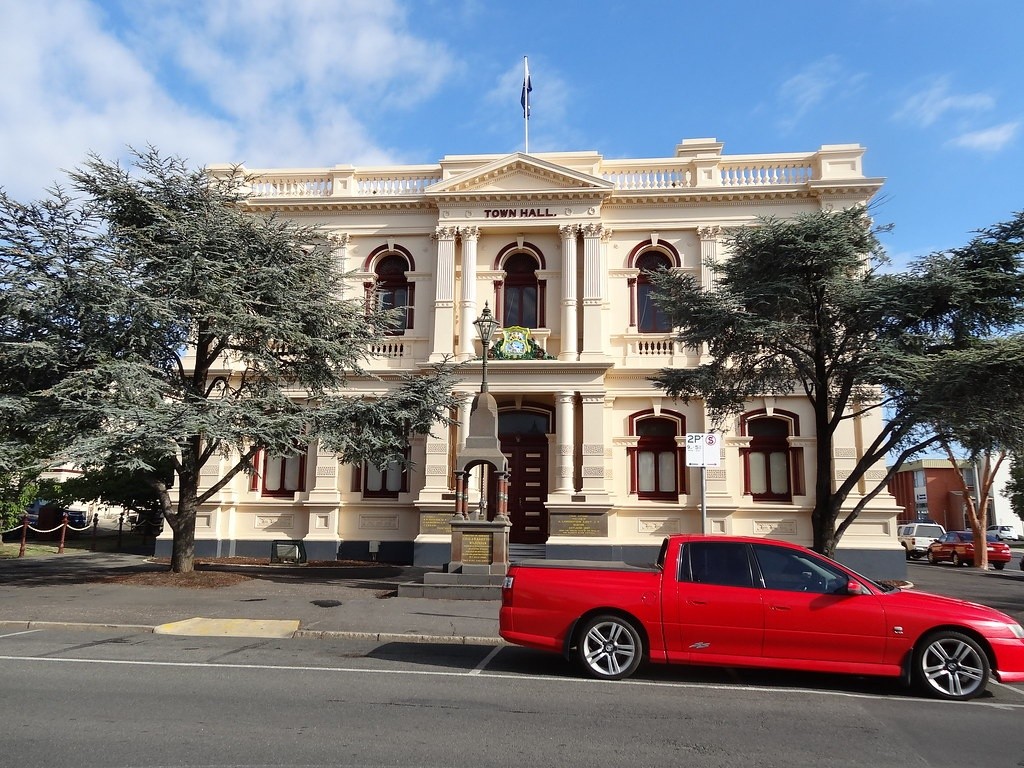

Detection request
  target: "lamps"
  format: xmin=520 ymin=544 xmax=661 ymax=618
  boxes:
xmin=277 ymin=544 xmax=301 ymax=565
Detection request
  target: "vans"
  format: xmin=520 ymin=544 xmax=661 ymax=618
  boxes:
xmin=896 ymin=523 xmax=948 ymax=560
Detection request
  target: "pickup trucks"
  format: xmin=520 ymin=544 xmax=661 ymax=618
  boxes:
xmin=497 ymin=535 xmax=1024 ymax=701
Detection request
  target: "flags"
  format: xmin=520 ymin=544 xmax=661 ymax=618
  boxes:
xmin=522 ymin=65 xmax=533 ymax=118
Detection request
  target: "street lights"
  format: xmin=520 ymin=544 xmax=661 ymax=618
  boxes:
xmin=471 ymin=299 xmax=500 ymax=393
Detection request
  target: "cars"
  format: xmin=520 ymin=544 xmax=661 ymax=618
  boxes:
xmin=926 ymin=530 xmax=1012 ymax=571
xmin=985 ymin=525 xmax=1019 ymax=542
